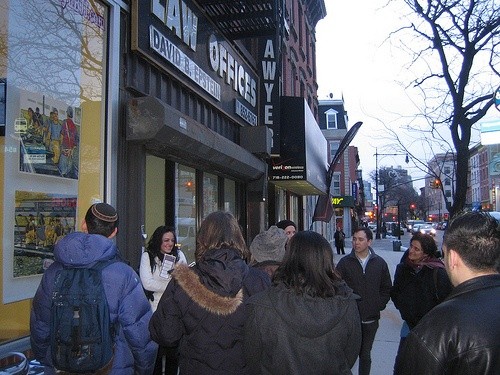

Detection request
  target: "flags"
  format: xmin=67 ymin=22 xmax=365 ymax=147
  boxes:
xmin=313 ymin=121 xmax=365 ymax=223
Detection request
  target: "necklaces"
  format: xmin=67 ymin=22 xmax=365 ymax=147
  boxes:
xmin=417 ymin=256 xmax=425 ymax=268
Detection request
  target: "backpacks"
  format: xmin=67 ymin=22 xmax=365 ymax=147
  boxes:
xmin=50 ymin=257 xmax=119 ymax=375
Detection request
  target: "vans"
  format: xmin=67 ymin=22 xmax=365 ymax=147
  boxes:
xmin=10 ymin=117 xmax=27 ymax=137
xmin=177 ymin=218 xmax=202 ymax=255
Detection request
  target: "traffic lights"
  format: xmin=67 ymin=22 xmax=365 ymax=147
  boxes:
xmin=430 ymin=180 xmax=435 ymax=189
xmin=435 ymin=180 xmax=440 ymax=189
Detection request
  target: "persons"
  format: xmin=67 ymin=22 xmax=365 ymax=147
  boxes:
xmin=243 ymin=230 xmax=362 ymax=375
xmin=381 ymin=223 xmax=387 ymax=238
xmin=148 ymin=211 xmax=274 ymax=374
xmin=392 ymin=211 xmax=500 ymax=374
xmin=29 ymin=201 xmax=158 ymax=375
xmin=393 ymin=232 xmax=454 ymax=356
xmin=138 ymin=225 xmax=188 ymax=315
xmin=333 ymin=225 xmax=393 ymax=375
xmin=333 ymin=225 xmax=346 ymax=254
xmin=248 ymin=225 xmax=288 ymax=280
xmin=276 ymin=221 xmax=297 ymax=246
xmin=365 ymin=223 xmax=369 ymax=231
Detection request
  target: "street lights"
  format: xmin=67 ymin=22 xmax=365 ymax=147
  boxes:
xmin=374 ymin=146 xmax=410 ymax=239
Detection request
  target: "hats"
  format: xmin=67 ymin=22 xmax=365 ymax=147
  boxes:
xmin=276 ymin=219 xmax=297 ymax=231
xmin=250 ymin=225 xmax=289 ymax=264
xmin=91 ymin=202 xmax=118 ymax=223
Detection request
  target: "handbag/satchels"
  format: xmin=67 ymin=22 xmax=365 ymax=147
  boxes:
xmin=132 ymin=266 xmax=155 ymax=302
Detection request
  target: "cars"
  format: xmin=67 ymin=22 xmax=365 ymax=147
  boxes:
xmin=363 ymin=220 xmax=449 ymax=238
